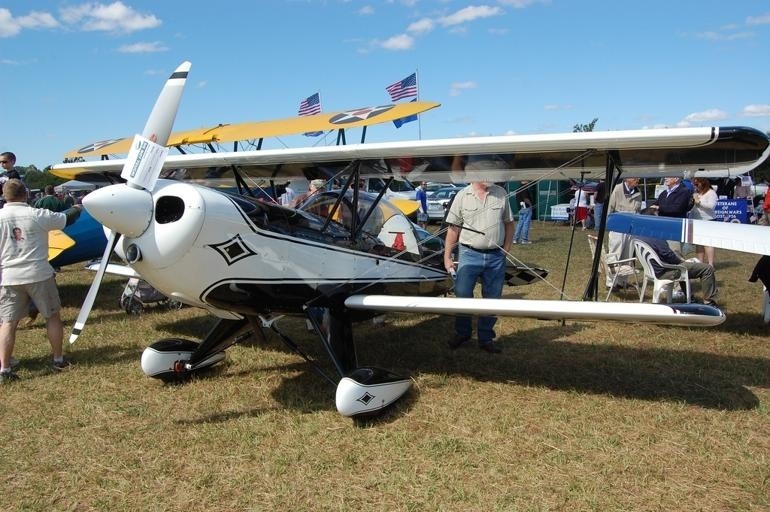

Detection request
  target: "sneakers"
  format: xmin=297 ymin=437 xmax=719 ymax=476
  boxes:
xmin=480 ymin=341 xmax=501 ymax=353
xmin=607 ymin=283 xmax=635 ymax=292
xmin=448 ymin=334 xmax=470 ymax=346
xmin=0 ymin=358 xmax=20 ymax=384
xmin=513 ymin=240 xmax=532 ymax=245
xmin=684 ymin=295 xmax=721 ymax=309
xmin=52 ymin=356 xmax=74 ymax=370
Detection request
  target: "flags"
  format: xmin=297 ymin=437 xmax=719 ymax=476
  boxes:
xmin=387 ymin=73 xmax=417 ymax=103
xmin=393 ymin=98 xmax=418 ymax=129
xmin=303 ymin=132 xmax=323 ymax=137
xmin=299 ymin=93 xmax=321 ymax=116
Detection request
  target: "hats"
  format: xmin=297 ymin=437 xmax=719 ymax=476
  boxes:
xmin=311 ymin=179 xmax=327 ymax=190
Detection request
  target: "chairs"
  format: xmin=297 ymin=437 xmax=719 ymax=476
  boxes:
xmin=588 ymin=234 xmax=692 ymax=303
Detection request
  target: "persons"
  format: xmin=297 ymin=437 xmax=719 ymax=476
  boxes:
xmin=0 ymin=152 xmax=104 ymax=381
xmin=416 ymin=182 xmax=427 ymax=225
xmin=442 ymin=163 xmax=514 ymax=353
xmin=576 ymin=174 xmax=770 ymax=323
xmin=513 ymin=178 xmax=535 ymax=245
xmin=257 ymin=175 xmax=369 ymax=230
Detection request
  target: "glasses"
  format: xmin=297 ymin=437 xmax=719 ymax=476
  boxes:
xmin=0 ymin=160 xmax=9 ymax=164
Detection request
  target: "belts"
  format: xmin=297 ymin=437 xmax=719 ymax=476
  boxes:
xmin=461 ymin=243 xmax=503 ymax=253
